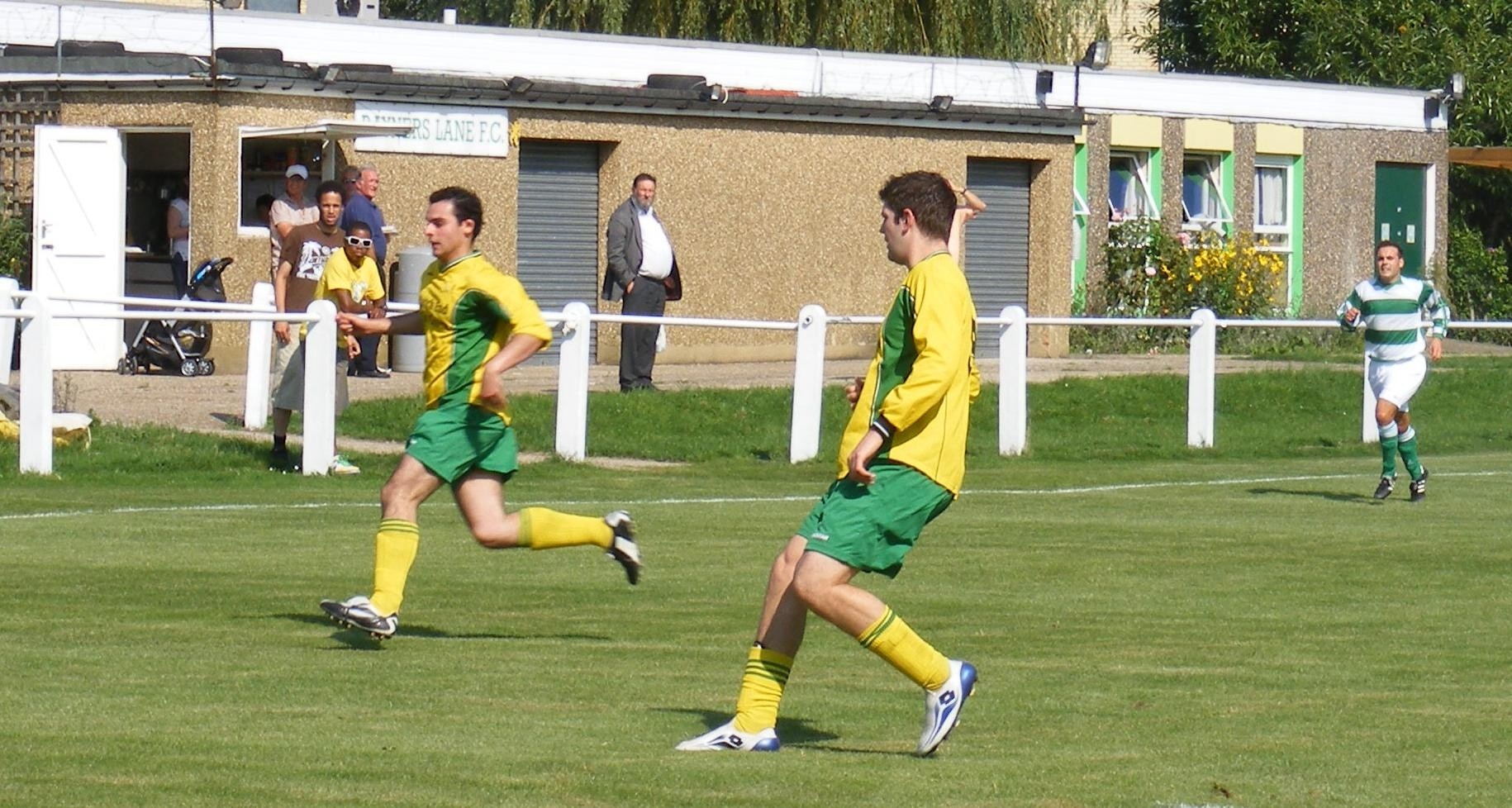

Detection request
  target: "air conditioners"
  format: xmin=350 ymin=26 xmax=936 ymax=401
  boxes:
xmin=306 ymin=0 xmax=379 ymax=20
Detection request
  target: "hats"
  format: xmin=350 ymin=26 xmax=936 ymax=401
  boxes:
xmin=285 ymin=164 xmax=308 ymax=179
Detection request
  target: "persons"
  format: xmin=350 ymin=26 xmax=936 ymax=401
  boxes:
xmin=675 ymin=168 xmax=988 ymax=758
xmin=319 ymin=185 xmax=640 ymax=643
xmin=1334 ymin=240 xmax=1450 ymax=502
xmin=268 ymin=180 xmax=346 ymax=473
xmin=300 ymin=221 xmax=389 ymax=475
xmin=167 ymin=177 xmax=190 ymax=299
xmin=600 ymin=172 xmax=683 ymax=395
xmin=256 ymin=164 xmax=391 ymax=378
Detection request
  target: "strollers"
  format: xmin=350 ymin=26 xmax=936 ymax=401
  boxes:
xmin=117 ymin=257 xmax=234 ymax=376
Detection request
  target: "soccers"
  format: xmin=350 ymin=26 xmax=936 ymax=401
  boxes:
xmin=343 ymin=595 xmax=369 ymax=605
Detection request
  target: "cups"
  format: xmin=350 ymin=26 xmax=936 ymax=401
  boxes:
xmin=160 ymin=187 xmax=168 ymax=199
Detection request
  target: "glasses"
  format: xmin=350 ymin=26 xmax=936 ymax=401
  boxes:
xmin=343 ymin=178 xmax=356 ymax=183
xmin=344 ymin=236 xmax=372 ymax=248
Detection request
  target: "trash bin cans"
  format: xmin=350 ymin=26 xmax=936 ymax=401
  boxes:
xmin=390 ymin=247 xmax=437 ymax=374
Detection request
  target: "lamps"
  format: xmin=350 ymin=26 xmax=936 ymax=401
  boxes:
xmin=700 ymin=84 xmax=722 ymax=105
xmin=507 ymin=77 xmax=534 ymax=94
xmin=930 ymin=96 xmax=953 ymax=112
xmin=317 ymin=65 xmax=343 ymax=84
xmin=1439 ymin=72 xmax=1466 ymax=101
xmin=1074 ymin=40 xmax=1111 ymax=106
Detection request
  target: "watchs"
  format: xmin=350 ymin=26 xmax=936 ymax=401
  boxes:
xmin=960 ymin=186 xmax=967 ymax=193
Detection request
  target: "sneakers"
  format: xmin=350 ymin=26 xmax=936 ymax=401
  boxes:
xmin=358 ymin=369 xmax=391 ymax=378
xmin=1375 ymin=471 xmax=1399 ymax=498
xmin=270 ymin=450 xmax=291 ymax=469
xmin=319 ymin=593 xmax=399 ymax=639
xmin=676 ymin=720 xmax=780 ymax=755
xmin=916 ymin=658 xmax=977 ymax=758
xmin=603 ymin=511 xmax=641 ymax=584
xmin=330 ymin=454 xmax=360 ymax=474
xmin=347 ymin=369 xmax=358 ymax=376
xmin=1410 ymin=464 xmax=1428 ymax=503
